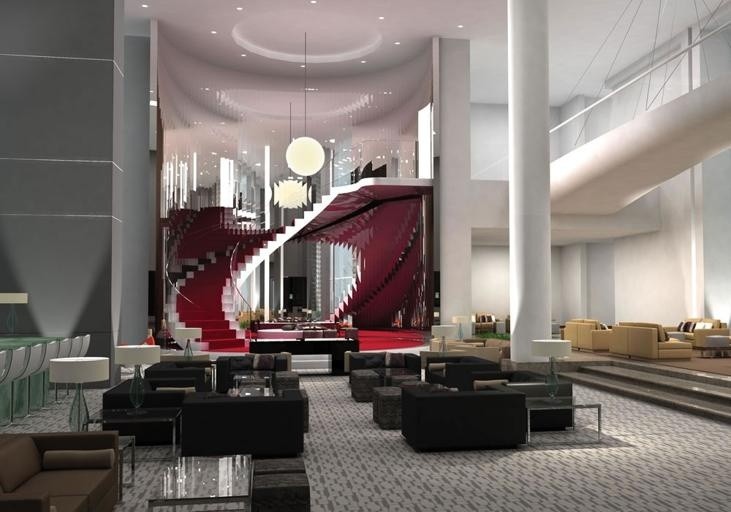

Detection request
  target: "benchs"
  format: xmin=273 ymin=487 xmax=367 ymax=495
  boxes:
xmin=257 ymin=328 xmax=303 ymax=338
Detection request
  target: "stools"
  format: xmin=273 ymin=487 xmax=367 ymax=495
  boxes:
xmin=253 ymin=474 xmax=310 ymax=511
xmin=253 ymin=460 xmax=306 ymax=473
xmin=303 ymin=327 xmax=359 ymax=338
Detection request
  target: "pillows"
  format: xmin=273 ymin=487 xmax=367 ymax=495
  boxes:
xmin=43 ymin=449 xmax=114 ymax=470
xmin=0 ymin=437 xmax=41 ymax=492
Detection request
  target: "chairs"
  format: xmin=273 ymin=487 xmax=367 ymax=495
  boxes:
xmin=102 ymin=338 xmax=572 ymax=459
xmin=0 ymin=333 xmax=91 ymax=427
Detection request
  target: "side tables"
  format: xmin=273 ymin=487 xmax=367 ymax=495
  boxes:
xmin=119 ymin=435 xmax=136 ymax=501
xmin=527 ymin=396 xmax=602 ymax=442
xmin=82 ymin=407 xmax=182 ymax=453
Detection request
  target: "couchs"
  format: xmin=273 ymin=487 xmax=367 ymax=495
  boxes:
xmin=560 ymin=318 xmax=731 ymax=362
xmin=0 ymin=430 xmax=119 ymax=512
xmin=476 ymin=311 xmax=495 ymax=328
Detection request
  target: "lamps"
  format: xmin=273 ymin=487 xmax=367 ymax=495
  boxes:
xmin=175 ymin=328 xmax=202 ymax=356
xmin=452 ymin=316 xmax=469 ymax=341
xmin=273 ymin=96 xmax=308 ymax=210
xmin=49 ymin=356 xmax=109 ymax=431
xmin=431 ymin=325 xmax=457 ymax=352
xmin=308 ymin=184 xmax=316 ymax=203
xmin=286 ymin=31 xmax=326 ymax=178
